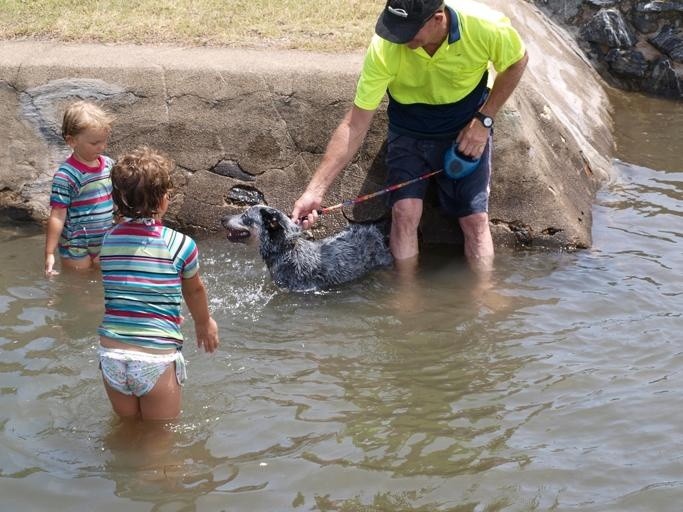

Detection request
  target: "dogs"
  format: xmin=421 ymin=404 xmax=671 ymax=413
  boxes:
xmin=221 ymin=204 xmax=399 ymax=295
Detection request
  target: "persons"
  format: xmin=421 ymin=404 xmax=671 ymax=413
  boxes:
xmin=94 ymin=141 xmax=219 ymax=423
xmin=285 ymin=2 xmax=535 ymax=324
xmin=39 ymin=97 xmax=123 ymax=281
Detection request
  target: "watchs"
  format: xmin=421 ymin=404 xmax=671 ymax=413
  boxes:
xmin=473 ymin=112 xmax=493 ymax=128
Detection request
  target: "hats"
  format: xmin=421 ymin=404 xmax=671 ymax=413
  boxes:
xmin=374 ymin=0 xmax=445 ymax=45
